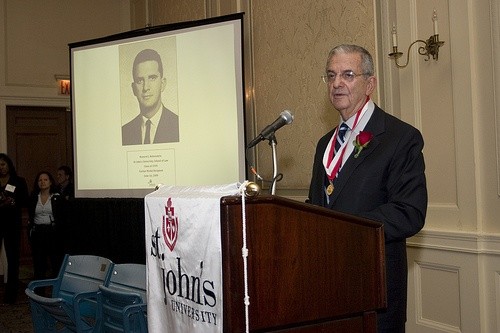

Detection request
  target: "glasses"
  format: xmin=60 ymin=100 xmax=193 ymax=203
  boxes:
xmin=324 ymin=72 xmax=371 ymax=80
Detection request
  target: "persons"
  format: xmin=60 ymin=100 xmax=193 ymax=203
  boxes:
xmin=0 ymin=153 xmax=28 ymax=302
xmin=27 ymin=170 xmax=67 ymax=297
xmin=122 ymin=49 xmax=179 ymax=146
xmin=304 ymin=44 xmax=428 ymax=333
xmin=55 ymin=166 xmax=74 ymax=203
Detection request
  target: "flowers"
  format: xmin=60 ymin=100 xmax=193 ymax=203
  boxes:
xmin=352 ymin=131 xmax=376 ymax=158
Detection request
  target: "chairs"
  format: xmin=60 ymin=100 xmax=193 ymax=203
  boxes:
xmin=25 ymin=254 xmax=148 ymax=333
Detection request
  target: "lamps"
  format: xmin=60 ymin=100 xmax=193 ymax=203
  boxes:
xmin=55 ymin=74 xmax=70 ymax=96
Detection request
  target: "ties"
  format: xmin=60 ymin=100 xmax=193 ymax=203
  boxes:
xmin=144 ymin=120 xmax=151 ymax=143
xmin=332 ymin=123 xmax=348 ymax=176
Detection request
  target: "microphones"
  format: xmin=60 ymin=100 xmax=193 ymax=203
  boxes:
xmin=248 ymin=110 xmax=294 ymax=148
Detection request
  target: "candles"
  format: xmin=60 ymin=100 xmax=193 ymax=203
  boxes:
xmin=433 ymin=9 xmax=438 ymax=34
xmin=391 ymin=22 xmax=397 ymax=46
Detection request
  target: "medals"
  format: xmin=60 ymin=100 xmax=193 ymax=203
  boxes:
xmin=327 ymin=185 xmax=333 ymax=195
